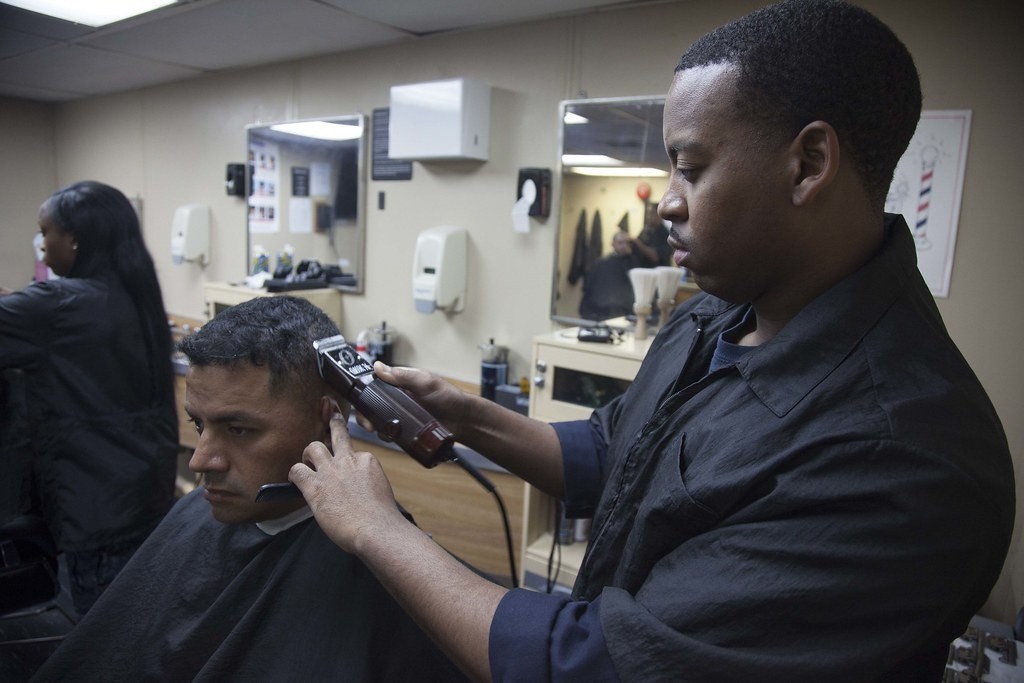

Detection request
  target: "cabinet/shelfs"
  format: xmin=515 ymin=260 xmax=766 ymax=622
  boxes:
xmin=209 ymin=284 xmax=340 ymax=333
xmin=520 ymin=325 xmax=656 ymax=595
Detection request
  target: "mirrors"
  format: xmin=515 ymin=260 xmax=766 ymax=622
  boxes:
xmin=247 ymin=112 xmax=366 ymax=293
xmin=548 ymin=97 xmax=702 ymax=334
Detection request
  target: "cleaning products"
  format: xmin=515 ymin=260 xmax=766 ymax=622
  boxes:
xmin=356 ymin=328 xmax=371 ymax=366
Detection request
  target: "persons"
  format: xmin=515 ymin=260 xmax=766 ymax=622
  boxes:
xmin=0 ymin=285 xmax=41 ymax=526
xmin=579 ymin=230 xmax=658 ymax=322
xmin=28 ymin=295 xmax=504 ymax=683
xmin=288 ymin=1 xmax=1016 ymax=683
xmin=628 ymin=202 xmax=676 ymax=268
xmin=0 ymin=181 xmax=179 ymax=625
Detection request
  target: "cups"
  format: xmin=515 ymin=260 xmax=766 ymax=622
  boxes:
xmin=368 ymin=328 xmax=395 ymax=365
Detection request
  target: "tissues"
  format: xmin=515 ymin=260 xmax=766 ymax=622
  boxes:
xmin=512 ymin=167 xmax=551 ymax=234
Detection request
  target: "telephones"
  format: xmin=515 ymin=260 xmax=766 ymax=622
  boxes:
xmin=578 ymin=323 xmax=611 ymax=342
